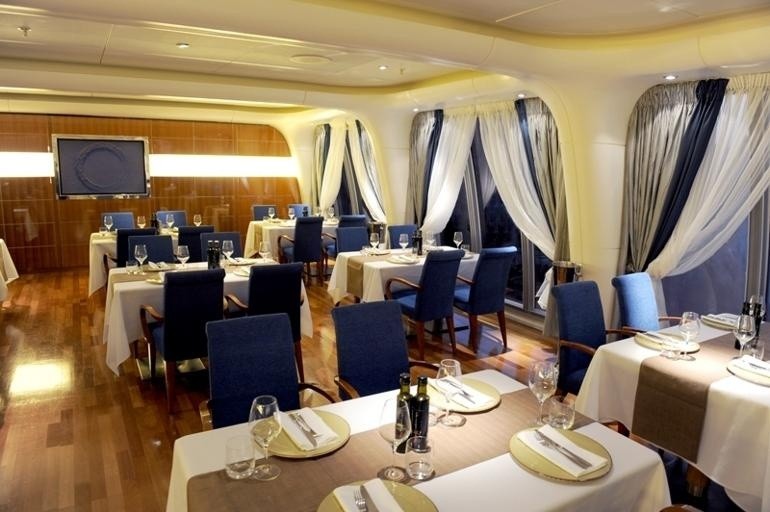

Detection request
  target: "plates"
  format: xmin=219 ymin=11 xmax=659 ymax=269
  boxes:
xmin=315 ymin=477 xmax=438 ymax=512
xmin=728 ymin=359 xmax=769 ymax=387
xmin=425 ymin=376 xmax=501 ymax=415
xmin=254 ymin=408 xmax=350 ymax=460
xmin=636 ymin=331 xmax=700 ymax=352
xmin=703 ymin=314 xmax=735 ymax=331
xmin=510 ymin=426 xmax=613 ymax=482
xmin=369 ymin=249 xmax=475 ymax=264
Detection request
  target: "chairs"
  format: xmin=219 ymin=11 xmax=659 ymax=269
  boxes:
xmin=253 ymin=205 xmax=277 ymax=219
xmin=199 ymin=231 xmax=242 ymax=261
xmin=548 ymin=280 xmax=685 ymax=500
xmin=103 ymin=227 xmax=157 ymax=288
xmin=386 ymin=248 xmax=466 ymax=360
xmin=451 ymin=244 xmax=518 ymax=354
xmin=333 ymin=299 xmax=443 ymax=398
xmin=101 ymin=212 xmax=133 ymax=230
xmin=323 ymin=214 xmax=367 ymax=279
xmin=277 ymin=216 xmax=324 ymax=287
xmin=127 ymin=234 xmax=177 ymax=264
xmin=335 ymin=226 xmax=370 ymax=253
xmin=611 ymin=272 xmax=683 ymax=341
xmin=154 ymin=210 xmax=188 ymax=227
xmin=387 ymin=224 xmax=418 ymax=248
xmin=199 ymin=313 xmax=342 ymax=432
xmin=226 ymin=260 xmax=306 ymax=383
xmin=140 ymin=268 xmax=227 ymax=413
xmin=287 ymin=202 xmax=312 ymax=217
xmin=177 ymin=225 xmax=214 ymax=262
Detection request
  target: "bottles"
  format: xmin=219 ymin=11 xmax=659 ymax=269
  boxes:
xmin=411 ymin=375 xmax=432 ymax=449
xmin=735 ymin=300 xmax=762 ymax=350
xmin=395 ymin=372 xmax=413 ymax=454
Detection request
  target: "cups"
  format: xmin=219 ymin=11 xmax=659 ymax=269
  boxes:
xmin=743 ymin=341 xmax=765 ymax=362
xmin=750 ymin=293 xmax=766 ymax=316
xmin=226 ymin=433 xmax=255 ymax=478
xmin=405 ymin=435 xmax=436 ymax=482
xmin=548 ymin=393 xmax=577 ymax=430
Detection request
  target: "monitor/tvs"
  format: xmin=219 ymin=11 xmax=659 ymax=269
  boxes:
xmin=50 ymin=133 xmax=151 ymax=200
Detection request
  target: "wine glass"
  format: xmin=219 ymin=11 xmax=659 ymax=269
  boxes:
xmin=434 ymin=357 xmax=470 ymax=425
xmin=526 ymin=361 xmax=558 ymax=426
xmin=248 ymin=396 xmax=283 ymax=483
xmin=376 ymin=397 xmax=409 ymax=480
xmin=425 ymin=231 xmax=436 ymax=247
xmin=370 ymin=233 xmax=380 ymax=249
xmin=399 ymin=232 xmax=410 ymax=252
xmin=453 ymin=231 xmax=464 ymax=248
xmin=678 ymin=310 xmax=700 ymax=360
xmin=102 ymin=203 xmax=337 ymax=276
xmin=732 ymin=313 xmax=756 ymax=357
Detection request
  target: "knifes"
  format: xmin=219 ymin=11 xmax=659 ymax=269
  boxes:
xmin=534 ymin=426 xmax=594 ymax=467
xmin=288 ymin=412 xmax=316 ymax=446
xmin=359 ymin=484 xmax=380 ymax=512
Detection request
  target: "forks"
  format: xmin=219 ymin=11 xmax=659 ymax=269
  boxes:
xmin=351 ymin=487 xmax=366 ymax=512
